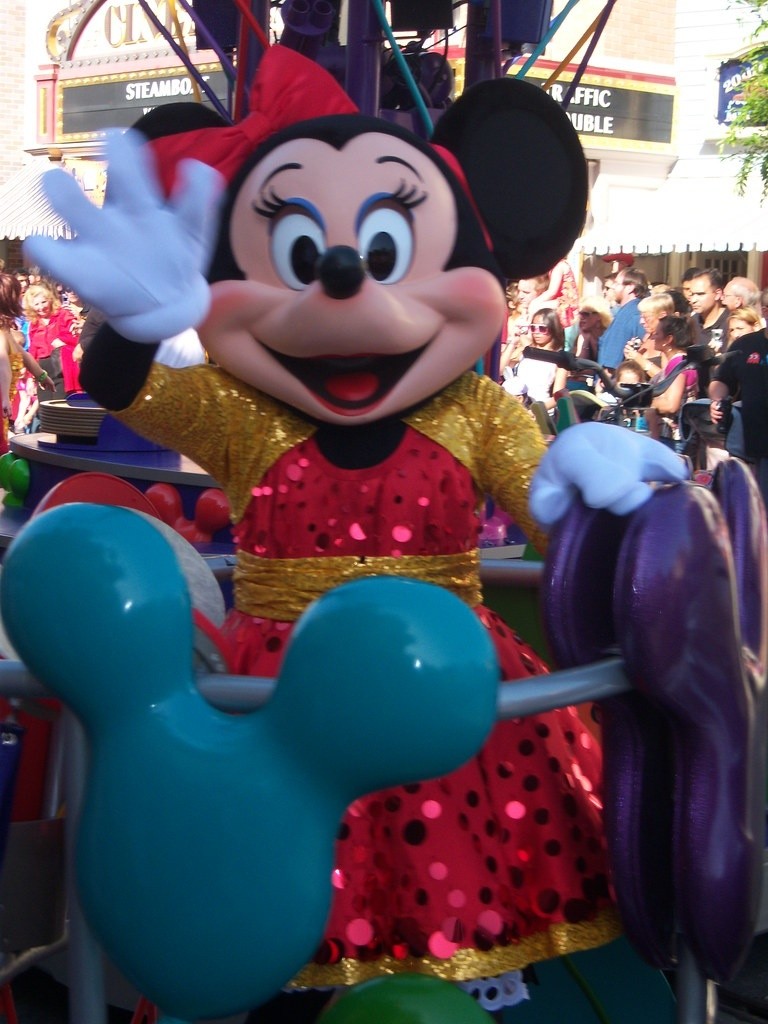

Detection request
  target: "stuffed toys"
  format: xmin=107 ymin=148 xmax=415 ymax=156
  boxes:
xmin=25 ymin=46 xmax=627 ymax=1024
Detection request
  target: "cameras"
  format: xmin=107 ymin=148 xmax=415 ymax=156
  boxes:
xmin=716 ymin=396 xmax=734 ymax=434
xmin=711 ymin=329 xmax=723 ymax=343
xmin=630 ymin=337 xmax=643 ymax=351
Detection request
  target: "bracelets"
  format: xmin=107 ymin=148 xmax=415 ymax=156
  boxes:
xmin=644 ymin=362 xmax=653 ymax=371
xmin=36 ymin=371 xmax=48 ymax=381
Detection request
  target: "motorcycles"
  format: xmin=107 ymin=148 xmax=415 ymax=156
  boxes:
xmin=520 ymin=343 xmax=740 ymax=455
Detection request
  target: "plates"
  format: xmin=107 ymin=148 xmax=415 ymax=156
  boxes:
xmin=39 ymin=400 xmax=108 ymax=436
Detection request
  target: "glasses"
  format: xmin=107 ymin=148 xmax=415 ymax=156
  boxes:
xmin=65 ymin=288 xmax=73 ymax=293
xmin=529 ymin=324 xmax=549 ymax=334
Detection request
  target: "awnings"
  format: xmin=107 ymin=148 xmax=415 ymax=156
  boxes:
xmin=0 ymin=160 xmax=102 ymax=240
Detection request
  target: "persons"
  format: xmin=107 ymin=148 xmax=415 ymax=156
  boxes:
xmin=0 ymin=260 xmax=107 ymax=455
xmin=496 ymin=255 xmax=768 ymax=501
